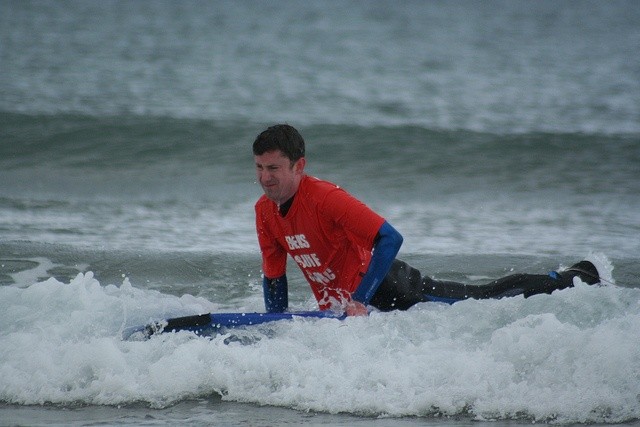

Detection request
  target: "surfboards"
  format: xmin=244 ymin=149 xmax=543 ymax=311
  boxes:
xmin=123 ymin=312 xmax=348 ymax=344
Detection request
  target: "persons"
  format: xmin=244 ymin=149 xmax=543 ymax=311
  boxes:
xmin=252 ymin=121 xmax=602 ymax=317
